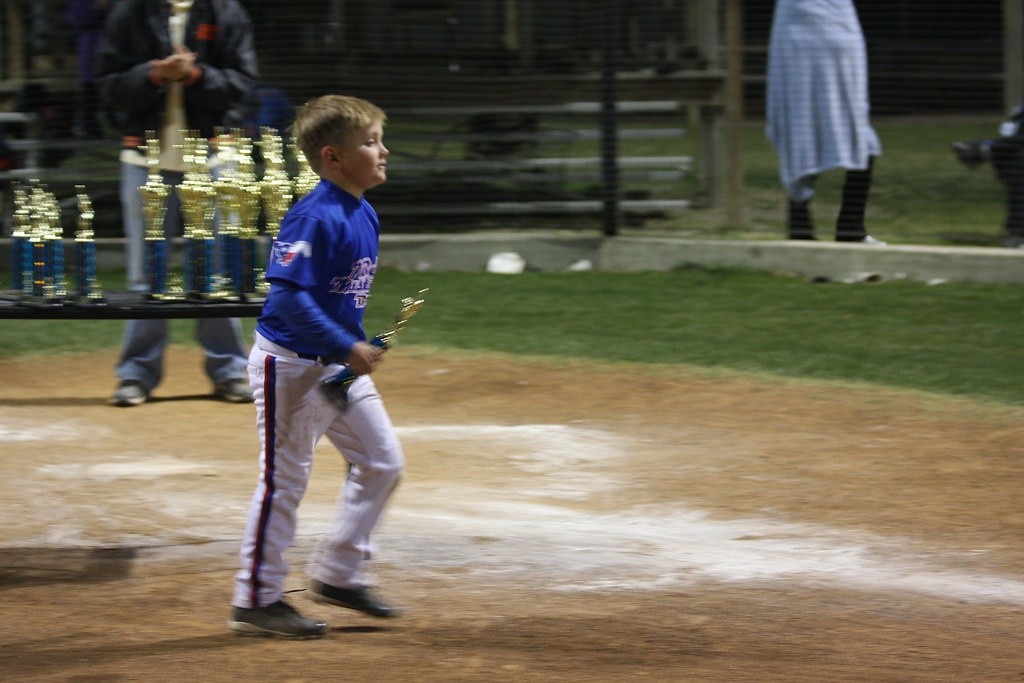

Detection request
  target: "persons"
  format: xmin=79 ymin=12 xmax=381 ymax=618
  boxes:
xmin=230 ymin=94 xmax=408 ymax=639
xmin=90 ymin=0 xmax=260 ymax=406
xmin=766 ymin=0 xmax=887 ymax=245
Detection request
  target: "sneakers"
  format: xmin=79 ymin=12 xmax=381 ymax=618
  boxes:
xmin=213 ymin=381 xmax=256 ymax=402
xmin=112 ymin=377 xmax=146 ymax=407
xmin=308 ymin=581 xmax=393 ymax=618
xmin=229 ymin=593 xmax=329 ymax=639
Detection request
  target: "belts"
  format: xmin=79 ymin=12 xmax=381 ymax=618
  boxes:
xmin=295 ymin=351 xmax=317 ymax=362
